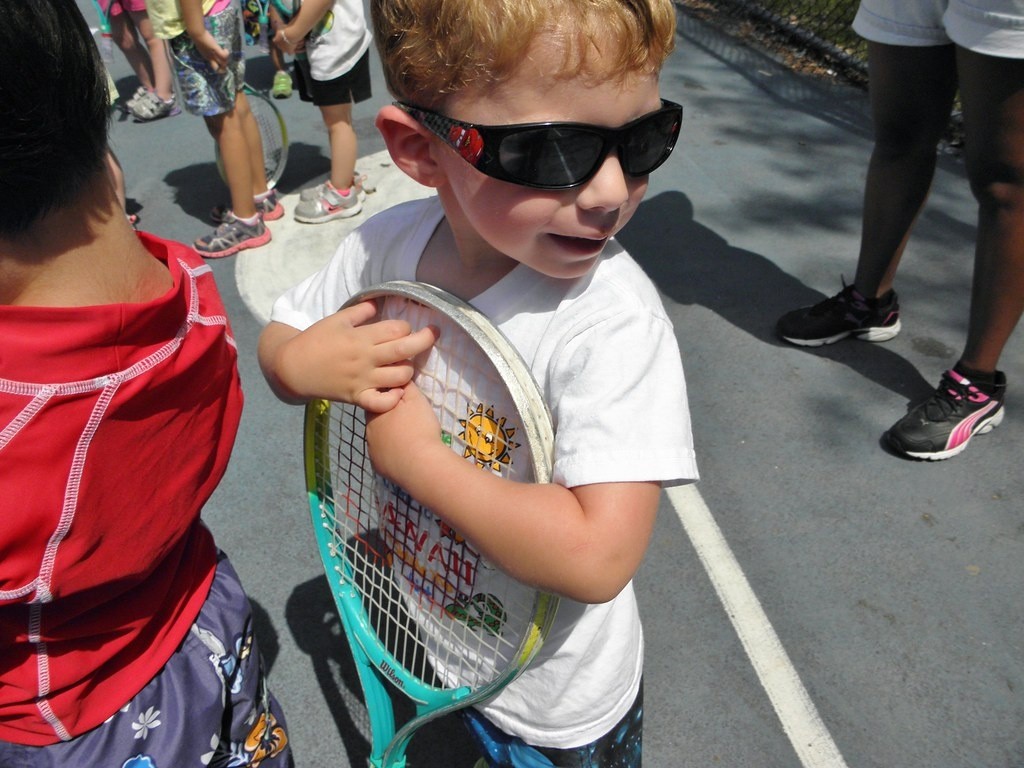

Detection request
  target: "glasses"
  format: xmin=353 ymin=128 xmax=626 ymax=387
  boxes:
xmin=393 ymin=98 xmax=684 ymax=190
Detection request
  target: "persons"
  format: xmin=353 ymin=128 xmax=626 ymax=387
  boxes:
xmin=95 ymin=0 xmax=374 ymax=260
xmin=774 ymin=0 xmax=1024 ymax=462
xmin=256 ymin=1 xmax=701 ymax=768
xmin=0 ymin=0 xmax=296 ymax=768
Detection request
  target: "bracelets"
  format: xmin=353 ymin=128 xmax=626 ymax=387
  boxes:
xmin=280 ymin=30 xmax=298 ymax=47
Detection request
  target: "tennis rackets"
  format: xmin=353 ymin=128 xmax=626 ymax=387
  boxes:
xmin=304 ymin=279 xmax=553 ymax=768
xmin=216 ymin=77 xmax=288 ymax=198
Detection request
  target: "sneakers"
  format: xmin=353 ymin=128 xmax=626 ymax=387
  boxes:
xmin=774 ymin=271 xmax=901 ymax=346
xmin=294 ymin=185 xmax=363 ymax=223
xmin=272 ymin=70 xmax=292 ymax=98
xmin=192 ymin=211 xmax=273 ymax=258
xmin=209 ymin=189 xmax=284 ymax=221
xmin=885 ymin=362 xmax=1008 ymax=461
xmin=132 ymin=90 xmax=181 ymax=121
xmin=299 ymin=180 xmax=367 ymax=203
xmin=122 ymin=87 xmax=150 ymax=113
